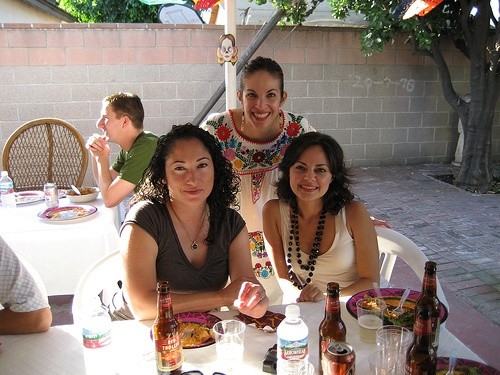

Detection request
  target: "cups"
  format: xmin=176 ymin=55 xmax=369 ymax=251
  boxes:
xmin=212 ymin=319 xmax=246 ymax=372
xmin=73 ymin=306 xmax=110 ymax=342
xmin=307 ymin=362 xmax=315 ymax=375
xmin=356 ymin=298 xmax=387 ymax=343
xmin=369 ymin=325 xmax=414 ymax=374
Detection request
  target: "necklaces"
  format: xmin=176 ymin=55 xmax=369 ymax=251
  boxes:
xmin=240 ymin=112 xmax=282 ymax=131
xmin=170 ymin=203 xmax=207 ymax=250
xmin=286 ymin=196 xmax=328 ymax=289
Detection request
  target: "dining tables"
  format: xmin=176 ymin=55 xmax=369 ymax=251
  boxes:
xmin=0 ymin=297 xmax=488 ymax=375
xmin=0 ymin=189 xmax=117 ymax=297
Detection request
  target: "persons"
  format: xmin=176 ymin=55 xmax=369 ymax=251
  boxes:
xmin=87 ymin=92 xmax=161 ymax=209
xmin=197 ymin=56 xmax=392 ymax=303
xmin=262 ymin=132 xmax=388 ymax=303
xmin=0 ymin=234 xmax=52 ymax=334
xmin=119 ymin=123 xmax=270 ymax=319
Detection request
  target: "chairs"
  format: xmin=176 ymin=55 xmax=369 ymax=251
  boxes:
xmin=374 ymin=223 xmax=450 ymax=316
xmin=0 ymin=117 xmax=88 ymax=192
xmin=71 ymin=249 xmax=134 ymax=323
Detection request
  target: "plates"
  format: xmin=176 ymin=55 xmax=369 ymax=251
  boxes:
xmin=150 ymin=312 xmax=227 ymax=349
xmin=347 ymin=288 xmax=449 ymax=333
xmin=38 ymin=204 xmax=98 ymax=222
xmin=435 ymin=354 xmax=499 ymax=374
xmin=0 ymin=186 xmax=65 ymax=205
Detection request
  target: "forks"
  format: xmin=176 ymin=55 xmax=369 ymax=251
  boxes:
xmin=389 ymin=289 xmax=411 ymax=318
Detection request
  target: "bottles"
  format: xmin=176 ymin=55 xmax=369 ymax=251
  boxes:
xmin=152 ymin=280 xmax=183 ymax=375
xmin=408 ymin=260 xmax=442 ymax=375
xmin=318 ymin=282 xmax=347 ymax=375
xmin=0 ymin=170 xmax=17 ymax=212
xmin=81 ymin=295 xmax=117 ymax=375
xmin=277 ymin=304 xmax=310 ymax=375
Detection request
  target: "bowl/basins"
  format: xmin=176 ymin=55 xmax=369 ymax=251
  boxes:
xmin=65 ymin=187 xmax=101 ymax=202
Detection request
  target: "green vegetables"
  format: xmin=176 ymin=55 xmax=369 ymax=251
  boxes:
xmin=376 ymin=310 xmax=415 ymax=329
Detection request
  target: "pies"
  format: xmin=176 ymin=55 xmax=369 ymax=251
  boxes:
xmin=178 ymin=323 xmax=211 ymax=345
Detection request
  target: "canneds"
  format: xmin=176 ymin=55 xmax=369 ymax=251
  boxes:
xmin=323 ymin=341 xmax=356 ymax=375
xmin=44 ymin=182 xmax=59 ymax=207
